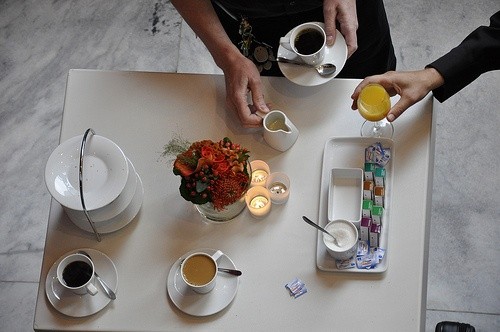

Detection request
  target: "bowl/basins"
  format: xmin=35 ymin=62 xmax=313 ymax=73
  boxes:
xmin=328 ymin=169 xmax=364 ymax=226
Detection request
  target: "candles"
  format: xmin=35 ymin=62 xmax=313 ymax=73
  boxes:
xmin=246 ymin=158 xmax=289 ymax=220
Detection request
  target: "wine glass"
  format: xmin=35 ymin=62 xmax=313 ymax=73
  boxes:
xmin=357 ymin=82 xmax=394 ymax=139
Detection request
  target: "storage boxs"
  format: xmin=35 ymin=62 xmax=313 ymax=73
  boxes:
xmin=328 ymin=167 xmax=365 ymax=224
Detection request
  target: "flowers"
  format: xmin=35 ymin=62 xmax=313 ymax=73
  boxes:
xmin=163 ymin=132 xmax=254 ymax=210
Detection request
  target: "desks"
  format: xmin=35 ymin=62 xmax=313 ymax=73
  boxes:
xmin=32 ymin=68 xmax=435 ymax=331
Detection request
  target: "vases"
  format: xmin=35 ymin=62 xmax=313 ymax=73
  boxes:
xmin=192 ymin=162 xmax=256 ymax=222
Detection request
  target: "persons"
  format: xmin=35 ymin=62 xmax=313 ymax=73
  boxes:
xmin=170 ymin=0 xmax=397 ymax=128
xmin=350 ymin=10 xmax=500 ymax=122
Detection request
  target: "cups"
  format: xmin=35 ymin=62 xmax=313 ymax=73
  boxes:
xmin=279 ymin=23 xmax=327 ymax=66
xmin=322 ymin=219 xmax=359 ymax=261
xmin=56 ymin=253 xmax=98 ymax=296
xmin=248 ymin=160 xmax=270 ymax=188
xmin=180 ymin=249 xmax=224 ymax=295
xmin=266 ymin=172 xmax=290 ymax=206
xmin=245 ymin=186 xmax=272 ymax=220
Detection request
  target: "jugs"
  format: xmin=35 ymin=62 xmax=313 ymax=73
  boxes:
xmin=254 ymin=110 xmax=299 ymax=152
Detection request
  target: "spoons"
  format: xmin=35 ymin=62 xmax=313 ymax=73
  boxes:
xmin=276 ymin=57 xmax=336 ymax=77
xmin=302 ymin=216 xmax=344 ymax=248
xmin=78 ymin=250 xmax=116 ymax=300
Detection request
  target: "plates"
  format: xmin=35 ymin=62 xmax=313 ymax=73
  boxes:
xmin=45 ymin=135 xmax=144 ymax=234
xmin=166 ymin=249 xmax=239 ymax=317
xmin=316 ymin=136 xmax=394 ymax=272
xmin=277 ymin=21 xmax=348 ymax=87
xmin=45 ymin=247 xmax=118 ymax=318
